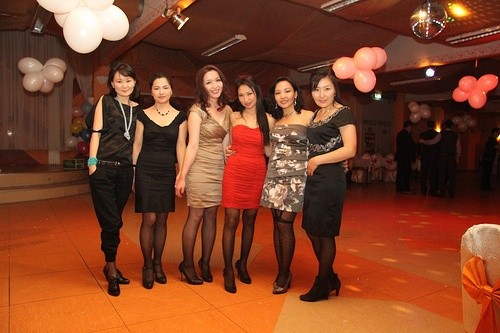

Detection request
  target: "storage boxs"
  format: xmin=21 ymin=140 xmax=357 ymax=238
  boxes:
xmin=64 ymin=159 xmax=84 ymax=171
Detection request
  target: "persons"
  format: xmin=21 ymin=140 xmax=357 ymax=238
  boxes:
xmin=299 ymin=71 xmax=359 ymax=302
xmin=87 ymin=61 xmax=142 ymax=296
xmin=132 ymin=72 xmax=188 ymax=290
xmin=393 ymin=119 xmax=500 ymax=198
xmin=221 ymin=75 xmax=277 ymax=293
xmin=175 ymin=64 xmax=233 ymax=284
xmin=225 ymin=75 xmax=348 ymax=294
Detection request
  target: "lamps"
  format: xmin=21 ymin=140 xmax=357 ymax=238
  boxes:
xmin=424 ymin=66 xmax=437 ymax=78
xmin=445 ymin=25 xmax=500 ymax=45
xmin=30 ymin=4 xmax=54 ymax=34
xmin=297 ymin=59 xmax=337 ymax=72
xmin=321 ymin=0 xmax=358 ymax=14
xmin=409 ymin=0 xmax=448 ymax=40
xmin=370 ymin=90 xmax=381 ymax=103
xmin=163 ymin=1 xmax=189 ymax=31
xmin=201 ymin=34 xmax=248 ymax=58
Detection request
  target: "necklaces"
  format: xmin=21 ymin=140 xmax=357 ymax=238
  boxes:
xmin=155 ymin=105 xmax=171 ymax=116
xmin=116 ymin=97 xmax=132 ymax=139
xmin=283 ymin=111 xmax=296 ymax=119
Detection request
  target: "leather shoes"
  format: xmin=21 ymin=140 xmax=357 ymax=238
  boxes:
xmin=102 ymin=265 xmax=130 ymax=297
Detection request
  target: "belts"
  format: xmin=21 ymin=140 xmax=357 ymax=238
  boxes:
xmin=98 ymin=161 xmax=122 ymax=166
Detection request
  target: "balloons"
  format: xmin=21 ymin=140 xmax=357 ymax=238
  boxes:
xmin=408 ymin=102 xmax=431 ymax=123
xmin=66 ymin=93 xmax=91 ymax=155
xmin=35 ymin=0 xmax=129 ymax=54
xmin=17 ymin=57 xmax=66 ymax=93
xmin=452 ymin=74 xmax=498 ymax=108
xmin=333 ymin=47 xmax=387 ymax=92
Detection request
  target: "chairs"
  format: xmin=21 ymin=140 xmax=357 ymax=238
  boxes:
xmin=351 ymin=154 xmax=397 ymax=184
xmin=460 ymin=224 xmax=500 ymax=333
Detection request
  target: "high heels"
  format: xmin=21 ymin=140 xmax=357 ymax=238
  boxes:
xmin=197 ymin=259 xmax=212 ymax=283
xmin=222 ymin=267 xmax=237 ymax=293
xmin=142 ymin=258 xmax=166 ymax=289
xmin=299 ymin=273 xmax=341 ymax=301
xmin=272 ymin=271 xmax=292 ymax=295
xmin=235 ymin=259 xmax=251 ymax=284
xmin=179 ymin=261 xmax=203 ymax=285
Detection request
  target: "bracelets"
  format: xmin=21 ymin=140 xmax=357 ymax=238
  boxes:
xmin=132 ymin=164 xmax=136 ymax=167
xmin=88 ymin=157 xmax=98 ymax=166
